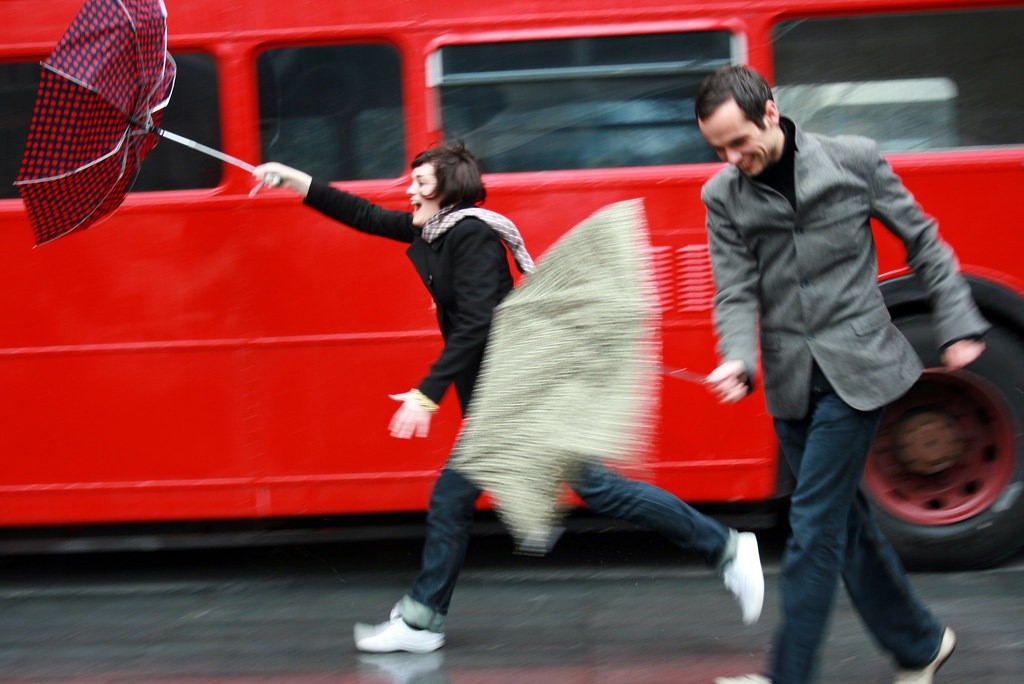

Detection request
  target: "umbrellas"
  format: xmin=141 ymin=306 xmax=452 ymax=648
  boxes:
xmin=14 ymin=0 xmax=280 ymax=245
xmin=447 ymin=198 xmax=709 ymax=552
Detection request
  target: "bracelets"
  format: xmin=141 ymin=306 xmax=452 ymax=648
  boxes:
xmin=411 ymin=388 xmax=440 ymax=412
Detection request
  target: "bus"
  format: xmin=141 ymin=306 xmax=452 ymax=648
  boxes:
xmin=0 ymin=3 xmax=1024 ymax=571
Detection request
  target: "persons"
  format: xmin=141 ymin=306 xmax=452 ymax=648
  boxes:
xmin=248 ymin=146 xmax=765 ymax=655
xmin=695 ymin=66 xmax=992 ymax=683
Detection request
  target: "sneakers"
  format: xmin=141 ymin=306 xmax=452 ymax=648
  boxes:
xmin=353 ymin=618 xmax=446 ymax=654
xmin=713 ymin=672 xmax=773 ymax=684
xmin=724 ymin=532 xmax=765 ymax=626
xmin=894 ymin=626 xmax=958 ymax=684
